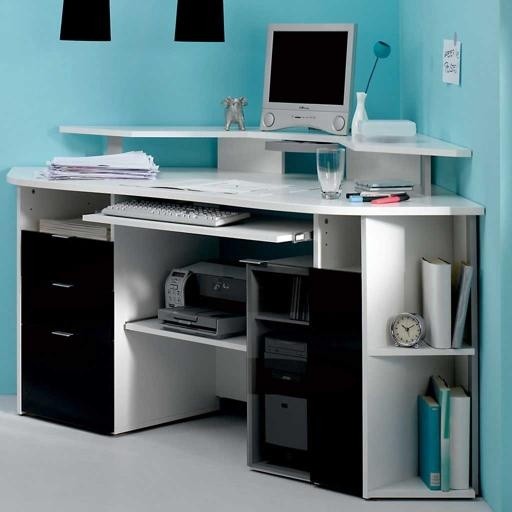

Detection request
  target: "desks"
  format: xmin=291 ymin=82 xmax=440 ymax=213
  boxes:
xmin=6 ymin=125 xmax=486 ymax=500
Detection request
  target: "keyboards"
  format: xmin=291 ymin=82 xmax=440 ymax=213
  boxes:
xmin=102 ymin=198 xmax=248 ymax=226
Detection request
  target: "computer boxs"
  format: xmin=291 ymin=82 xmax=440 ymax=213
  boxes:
xmin=257 ymin=332 xmax=317 ymax=472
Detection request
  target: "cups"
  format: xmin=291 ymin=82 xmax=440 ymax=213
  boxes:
xmin=315 ymin=146 xmax=346 ymax=202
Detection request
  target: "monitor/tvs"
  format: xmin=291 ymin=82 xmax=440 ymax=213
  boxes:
xmin=258 ymin=24 xmax=354 ymax=137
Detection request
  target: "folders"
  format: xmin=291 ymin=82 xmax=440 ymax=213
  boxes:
xmin=433 ymin=373 xmax=451 ymax=491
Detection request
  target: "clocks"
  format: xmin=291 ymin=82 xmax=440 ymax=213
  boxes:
xmin=388 ymin=311 xmax=426 ymax=349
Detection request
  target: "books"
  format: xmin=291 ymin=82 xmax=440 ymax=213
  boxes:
xmin=40 ymin=218 xmax=114 ymax=241
xmin=346 ymin=180 xmax=415 ymax=205
xmin=430 ymin=373 xmax=449 ymax=493
xmin=447 ymin=384 xmax=472 ymax=492
xmin=40 ymin=149 xmax=161 ymax=182
xmin=417 ymin=394 xmax=439 ymax=491
xmin=415 ymin=255 xmax=451 ymax=348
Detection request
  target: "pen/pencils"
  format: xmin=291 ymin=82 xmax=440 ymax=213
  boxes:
xmin=349 ymin=195 xmax=389 ymax=202
xmin=346 ymin=191 xmax=407 ymax=198
xmin=372 ymin=194 xmax=409 ymax=204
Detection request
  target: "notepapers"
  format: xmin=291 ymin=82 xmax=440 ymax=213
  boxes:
xmin=441 ymin=38 xmax=462 ymax=87
